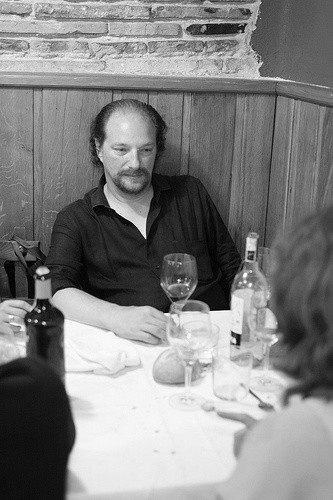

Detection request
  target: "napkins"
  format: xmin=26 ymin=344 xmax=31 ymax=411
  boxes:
xmin=61 ymin=328 xmax=142 ymax=377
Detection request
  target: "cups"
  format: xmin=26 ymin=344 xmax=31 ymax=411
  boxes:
xmin=212 ymin=346 xmax=252 ymax=399
xmin=182 ymin=321 xmax=219 ymax=371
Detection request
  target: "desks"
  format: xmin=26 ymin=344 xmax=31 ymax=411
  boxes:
xmin=0 ymin=306 xmax=305 ymax=500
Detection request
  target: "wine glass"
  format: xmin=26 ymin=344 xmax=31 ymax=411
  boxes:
xmin=167 ymin=300 xmax=213 ymax=407
xmin=160 ymin=253 xmax=197 ymax=328
xmin=246 ymin=279 xmax=281 ymax=355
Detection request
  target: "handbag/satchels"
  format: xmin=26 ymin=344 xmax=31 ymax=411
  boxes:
xmin=4 ymin=236 xmax=47 ymax=299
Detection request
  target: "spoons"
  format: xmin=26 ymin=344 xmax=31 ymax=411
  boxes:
xmin=240 ymin=384 xmax=274 ymax=409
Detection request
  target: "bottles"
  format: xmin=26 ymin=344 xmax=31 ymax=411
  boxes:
xmin=24 ymin=266 xmax=66 ymax=387
xmin=228 ymin=231 xmax=269 ymax=366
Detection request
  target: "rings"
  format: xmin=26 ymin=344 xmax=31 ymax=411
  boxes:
xmin=8 ymin=314 xmax=15 ymax=322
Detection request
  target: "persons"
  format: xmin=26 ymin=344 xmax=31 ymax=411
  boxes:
xmin=0 ymin=343 xmax=76 ymax=500
xmin=0 ymin=298 xmax=32 ymax=336
xmin=65 ymin=203 xmax=333 ymax=500
xmin=43 ymin=99 xmax=242 ymax=344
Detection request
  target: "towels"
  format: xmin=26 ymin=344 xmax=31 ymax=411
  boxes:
xmin=62 ymin=320 xmax=142 ymax=375
